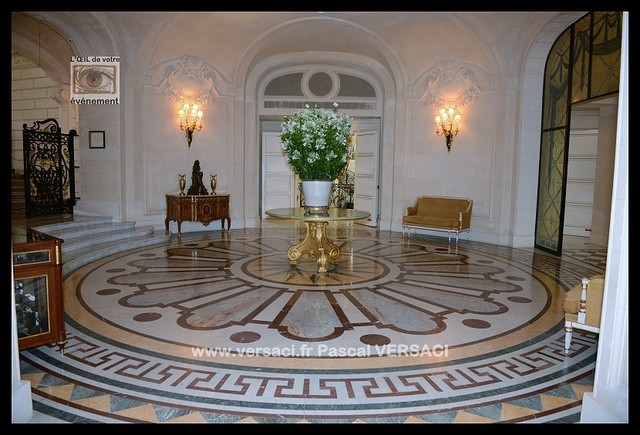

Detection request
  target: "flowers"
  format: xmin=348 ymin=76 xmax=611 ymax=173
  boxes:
xmin=278 ymin=99 xmax=356 ymax=182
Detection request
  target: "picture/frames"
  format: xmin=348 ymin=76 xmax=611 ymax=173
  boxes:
xmin=68 ymin=55 xmax=120 ymax=106
xmin=88 ymin=129 xmax=105 ymax=149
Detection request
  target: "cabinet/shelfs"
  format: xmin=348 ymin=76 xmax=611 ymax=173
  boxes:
xmin=11 ymin=222 xmax=69 ymax=355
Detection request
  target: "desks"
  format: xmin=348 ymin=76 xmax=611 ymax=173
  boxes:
xmin=265 ymin=206 xmax=372 ymax=274
xmin=163 ymin=193 xmax=231 ymax=242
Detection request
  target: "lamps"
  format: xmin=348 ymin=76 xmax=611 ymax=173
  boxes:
xmin=433 ymin=106 xmax=462 ymax=154
xmin=177 ymin=100 xmax=204 ymax=149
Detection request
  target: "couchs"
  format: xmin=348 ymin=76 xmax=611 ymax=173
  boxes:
xmin=401 ymin=195 xmax=474 ymax=246
xmin=561 ymin=271 xmax=605 ymax=354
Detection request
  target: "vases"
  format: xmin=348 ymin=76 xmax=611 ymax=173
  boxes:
xmin=301 ymin=180 xmax=333 ymax=209
xmin=210 ymin=174 xmax=217 ymax=195
xmin=178 ymin=173 xmax=186 ymax=196
